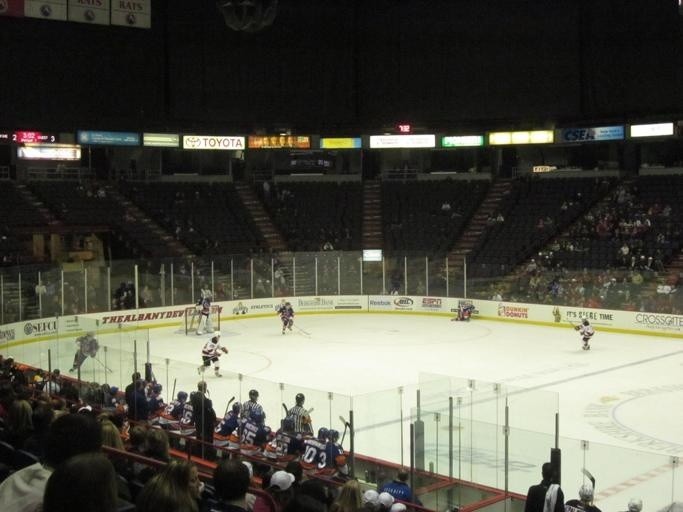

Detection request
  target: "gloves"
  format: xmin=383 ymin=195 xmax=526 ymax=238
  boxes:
xmin=214 ymin=351 xmax=220 ymax=356
xmin=221 ymin=347 xmax=227 ymax=353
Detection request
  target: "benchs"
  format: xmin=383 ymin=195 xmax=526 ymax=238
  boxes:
xmin=0 ymin=377 xmax=434 ymax=512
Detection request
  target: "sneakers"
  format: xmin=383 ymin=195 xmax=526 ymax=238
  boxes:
xmin=198 ymin=367 xmax=202 ymax=375
xmin=283 ymin=329 xmax=285 ymax=334
xmin=215 ymin=373 xmax=221 ymax=377
xmin=288 ymin=326 xmax=292 ymax=330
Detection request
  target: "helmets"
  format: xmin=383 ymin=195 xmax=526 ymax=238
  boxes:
xmin=87 ymin=331 xmax=94 ymax=340
xmin=152 ymin=383 xmax=196 ymax=404
xmin=582 ymin=316 xmax=586 ymax=323
xmin=282 ymin=394 xmax=338 ymax=440
xmin=578 ymin=483 xmax=593 ymax=501
xmin=213 ymin=331 xmax=221 ymax=337
xmin=231 ymin=390 xmax=265 ymax=422
xmin=398 ymin=467 xmax=409 ymax=481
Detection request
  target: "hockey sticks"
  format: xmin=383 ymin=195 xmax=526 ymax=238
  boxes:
xmin=562 ymin=315 xmax=575 ymax=329
xmin=77 ymin=345 xmax=112 ymax=373
xmin=201 ymin=350 xmax=224 ymax=365
xmin=287 ymin=317 xmax=313 ymax=336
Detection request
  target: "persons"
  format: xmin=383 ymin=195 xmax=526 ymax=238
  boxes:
xmin=575 ymin=316 xmax=593 ymax=350
xmin=441 ymin=200 xmax=450 ymax=213
xmin=69 ymin=332 xmax=99 ymax=373
xmin=564 ymin=484 xmax=602 ymax=512
xmin=1 ymin=180 xmax=332 ymax=316
xmin=0 ymin=354 xmax=423 ymax=511
xmin=478 ymin=180 xmax=681 ymax=315
xmin=456 ymin=301 xmax=474 ymax=321
xmin=196 ymin=291 xmax=214 ymax=334
xmin=198 ymin=331 xmax=228 ymax=377
xmin=524 ymin=462 xmax=565 ymax=512
xmin=278 ymin=302 xmax=294 ymax=334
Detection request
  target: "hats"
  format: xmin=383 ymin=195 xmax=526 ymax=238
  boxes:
xmin=269 ymin=469 xmax=295 ymax=491
xmin=77 ymin=405 xmax=92 ymax=413
xmin=362 ymin=489 xmax=407 ymax=512
